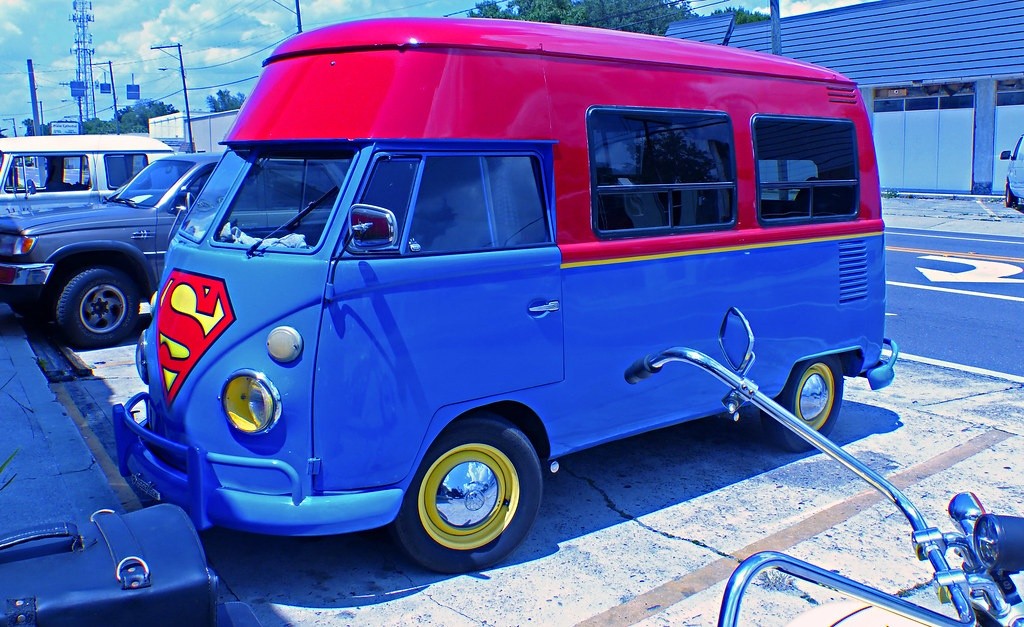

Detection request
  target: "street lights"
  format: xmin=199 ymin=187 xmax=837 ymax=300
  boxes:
xmin=27 ymin=101 xmax=45 ymax=136
xmin=157 ymin=66 xmax=193 ymax=153
xmin=3 ymin=118 xmax=18 ymax=137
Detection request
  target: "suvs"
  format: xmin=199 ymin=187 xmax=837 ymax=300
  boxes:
xmin=0 ymin=150 xmax=349 ymax=350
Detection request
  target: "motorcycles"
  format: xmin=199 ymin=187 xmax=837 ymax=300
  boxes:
xmin=621 ymin=306 xmax=1024 ymax=627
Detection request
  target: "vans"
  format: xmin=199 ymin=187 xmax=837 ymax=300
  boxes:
xmin=0 ymin=133 xmax=182 ymax=221
xmin=110 ymin=17 xmax=900 ymax=574
